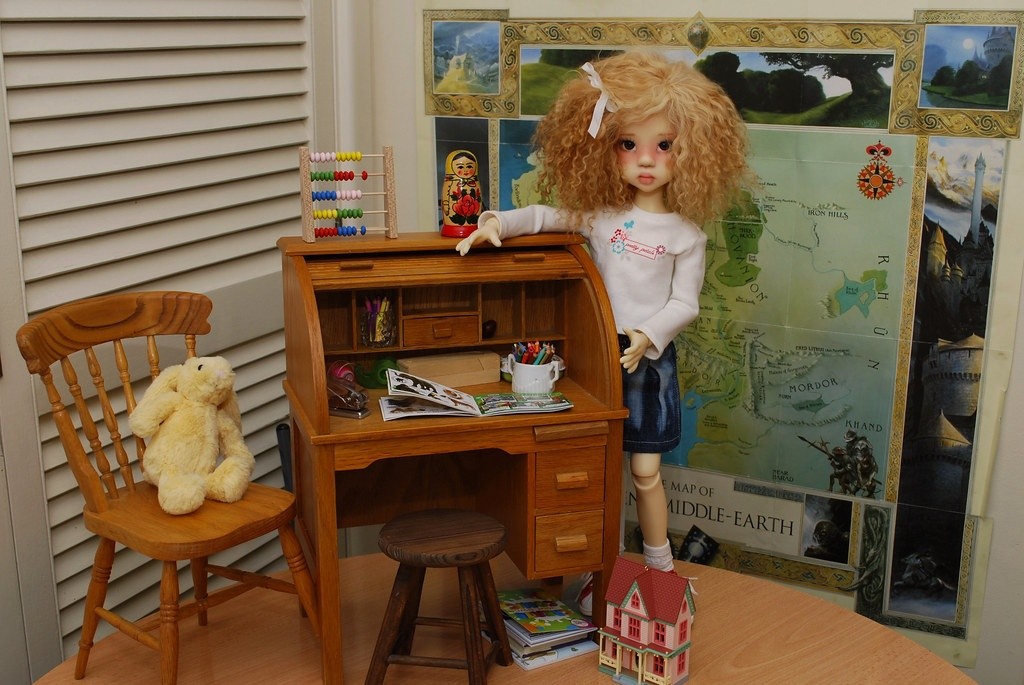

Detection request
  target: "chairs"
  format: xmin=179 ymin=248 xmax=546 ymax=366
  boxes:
xmin=15 ymin=291 xmax=320 ymax=685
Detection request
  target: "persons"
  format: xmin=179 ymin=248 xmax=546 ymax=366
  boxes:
xmin=456 ymin=49 xmax=751 ymax=577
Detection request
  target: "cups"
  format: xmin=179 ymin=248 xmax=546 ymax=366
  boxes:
xmin=361 ymin=311 xmax=395 ymax=347
xmin=509 ymin=355 xmax=559 ymax=394
xmin=679 ymin=525 xmax=720 ymax=564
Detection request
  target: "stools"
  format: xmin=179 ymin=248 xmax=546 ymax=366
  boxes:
xmin=364 ymin=509 xmax=514 ymax=685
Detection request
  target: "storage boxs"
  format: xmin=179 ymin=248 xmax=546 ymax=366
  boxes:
xmin=397 ymin=350 xmax=500 ymax=388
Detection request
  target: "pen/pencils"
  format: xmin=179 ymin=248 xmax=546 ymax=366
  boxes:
xmin=513 ymin=342 xmax=555 ymax=366
xmin=363 ymin=295 xmax=391 ymax=342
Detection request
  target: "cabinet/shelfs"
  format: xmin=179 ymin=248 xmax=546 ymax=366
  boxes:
xmin=277 ymin=231 xmax=630 ymax=685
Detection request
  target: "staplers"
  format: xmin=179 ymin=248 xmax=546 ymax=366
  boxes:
xmin=327 ymin=386 xmax=370 ymax=419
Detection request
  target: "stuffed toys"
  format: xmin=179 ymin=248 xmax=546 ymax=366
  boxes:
xmin=128 ymin=355 xmax=257 ymax=515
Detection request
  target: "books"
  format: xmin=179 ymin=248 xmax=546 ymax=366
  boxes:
xmin=478 ymin=586 xmax=600 ymax=670
xmin=378 ymin=368 xmax=574 ymax=421
